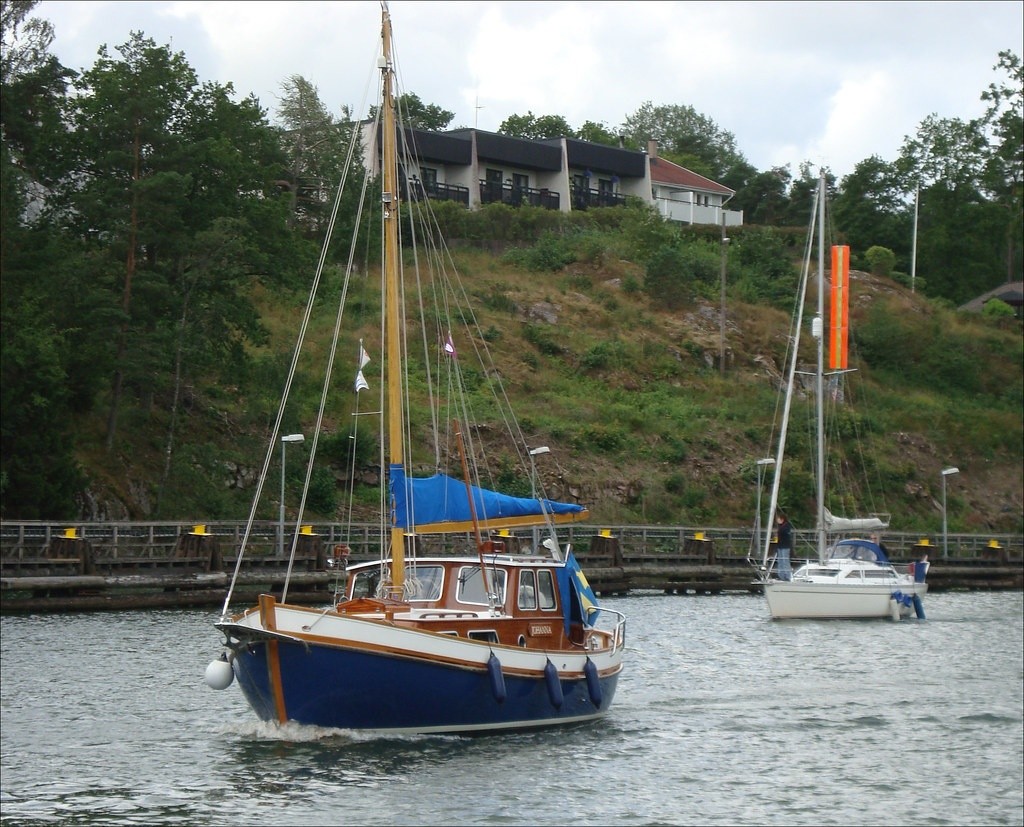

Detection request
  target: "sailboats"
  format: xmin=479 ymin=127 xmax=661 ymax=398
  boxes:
xmin=743 ymin=166 xmax=931 ymax=620
xmin=207 ymin=3 xmax=625 ymax=739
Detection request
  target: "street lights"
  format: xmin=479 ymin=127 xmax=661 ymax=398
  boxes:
xmin=529 ymin=444 xmax=552 ymax=557
xmin=756 ymin=457 xmax=776 ymax=556
xmin=278 ymin=433 xmax=307 ymax=562
xmin=941 ymin=468 xmax=959 ymax=560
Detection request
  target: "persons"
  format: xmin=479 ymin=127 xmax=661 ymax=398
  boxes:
xmin=776 ymin=514 xmax=792 ymax=581
